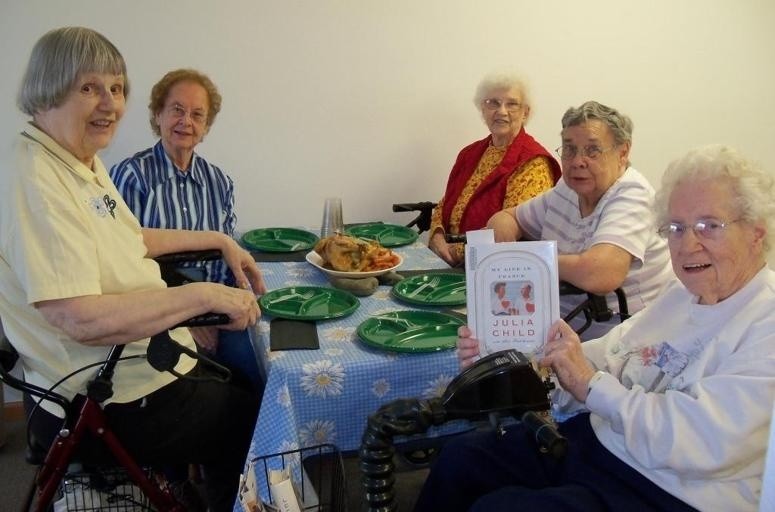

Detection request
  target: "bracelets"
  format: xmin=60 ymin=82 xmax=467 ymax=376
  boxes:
xmin=588 ymin=371 xmax=605 ymax=393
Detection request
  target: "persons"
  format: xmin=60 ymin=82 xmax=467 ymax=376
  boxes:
xmin=110 ymin=69 xmax=262 ymax=351
xmin=491 ymin=283 xmax=517 ymax=314
xmin=412 ymin=148 xmax=774 ymax=512
xmin=487 ymin=103 xmax=677 ymax=344
xmin=514 ymin=283 xmax=533 ymax=314
xmin=428 ymin=77 xmax=562 ymax=267
xmin=0 ymin=25 xmax=268 ymax=512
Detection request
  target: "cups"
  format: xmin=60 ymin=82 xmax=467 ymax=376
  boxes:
xmin=319 ymin=197 xmax=344 ymax=240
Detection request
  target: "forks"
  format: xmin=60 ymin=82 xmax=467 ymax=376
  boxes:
xmin=269 ymin=289 xmax=314 ymax=306
xmin=408 ymin=274 xmax=441 ymax=299
xmin=360 ymin=312 xmax=429 ymax=328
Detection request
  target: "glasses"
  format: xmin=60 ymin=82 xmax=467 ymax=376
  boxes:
xmin=483 ymin=98 xmax=523 ymax=111
xmin=554 ymin=144 xmax=613 ymax=160
xmin=657 ymin=219 xmax=737 ymax=237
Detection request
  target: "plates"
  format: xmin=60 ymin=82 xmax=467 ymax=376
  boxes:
xmin=394 ymin=271 xmax=467 ymax=307
xmin=357 ymin=309 xmax=466 ymax=353
xmin=344 ymin=223 xmax=419 ymax=248
xmin=241 ymin=227 xmax=320 ymax=255
xmin=259 ymin=286 xmax=360 ymax=320
xmin=306 ymin=250 xmax=404 ymax=281
